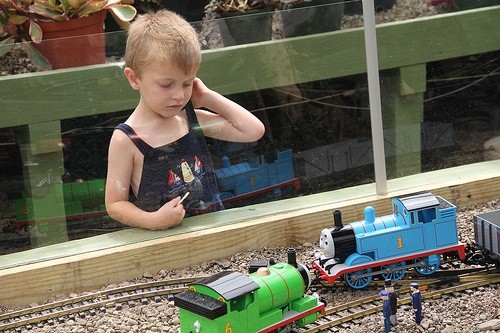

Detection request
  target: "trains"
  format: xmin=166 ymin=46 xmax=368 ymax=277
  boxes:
xmin=211 ymin=119 xmax=456 ymax=208
xmin=172 ymin=248 xmax=324 ymax=333
xmin=10 ymin=174 xmax=115 ymax=236
xmin=313 ymin=191 xmax=500 ymax=290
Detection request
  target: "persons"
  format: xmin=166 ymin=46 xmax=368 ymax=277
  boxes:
xmin=379 ymin=279 xmax=398 ymax=333
xmin=410 ymin=283 xmax=422 ymax=323
xmin=104 ymin=9 xmax=265 ymax=230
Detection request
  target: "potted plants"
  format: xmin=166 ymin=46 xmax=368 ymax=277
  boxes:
xmin=197 ymin=0 xmax=345 ymax=50
xmin=0 ymin=0 xmax=138 ymax=72
